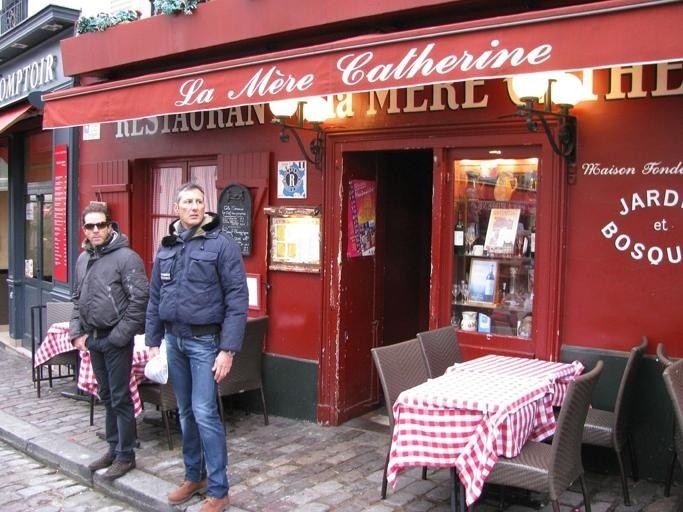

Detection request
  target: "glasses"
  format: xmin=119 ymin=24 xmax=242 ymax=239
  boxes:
xmin=84 ymin=222 xmax=109 ymax=229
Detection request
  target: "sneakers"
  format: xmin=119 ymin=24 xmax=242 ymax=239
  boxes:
xmin=103 ymin=452 xmax=135 ymax=479
xmin=89 ymin=452 xmax=117 ymax=471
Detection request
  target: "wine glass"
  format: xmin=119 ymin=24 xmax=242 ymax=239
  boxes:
xmin=452 ymin=279 xmax=471 ymax=305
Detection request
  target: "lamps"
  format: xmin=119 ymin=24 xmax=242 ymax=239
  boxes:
xmin=503 ymin=73 xmax=585 ymax=185
xmin=262 ymin=96 xmax=332 ymax=170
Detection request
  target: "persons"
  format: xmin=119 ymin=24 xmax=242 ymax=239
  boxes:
xmin=144 ymin=182 xmax=248 ymax=509
xmin=68 ymin=202 xmax=150 ymax=484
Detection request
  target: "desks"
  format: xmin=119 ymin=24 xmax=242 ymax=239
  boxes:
xmin=379 ymin=353 xmax=594 ymax=512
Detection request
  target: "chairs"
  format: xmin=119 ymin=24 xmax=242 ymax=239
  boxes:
xmin=414 ymin=326 xmax=461 ymax=481
xmin=654 ymin=342 xmax=683 ymax=512
xmin=370 ymin=334 xmax=461 ymax=511
xmin=549 ymin=334 xmax=647 ymax=507
xmin=23 ymin=300 xmax=271 ymax=450
xmin=459 ymin=358 xmax=605 ymax=512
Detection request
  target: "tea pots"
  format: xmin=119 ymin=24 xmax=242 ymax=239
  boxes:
xmin=462 ymin=171 xmax=485 ymax=202
xmin=460 ymin=311 xmax=477 ymax=332
xmin=492 ymin=171 xmax=518 ymax=202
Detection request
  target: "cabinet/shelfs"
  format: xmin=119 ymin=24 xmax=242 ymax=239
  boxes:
xmin=449 ymin=154 xmax=542 ymax=343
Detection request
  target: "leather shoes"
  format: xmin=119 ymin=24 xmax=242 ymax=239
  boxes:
xmin=198 ymin=497 xmax=231 ymax=512
xmin=168 ymin=478 xmax=208 ymax=505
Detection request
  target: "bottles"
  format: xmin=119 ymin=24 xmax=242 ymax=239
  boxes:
xmin=530 ymin=222 xmax=537 ymax=257
xmin=453 ymin=211 xmax=465 ymax=256
xmin=450 ymin=310 xmax=459 ymax=330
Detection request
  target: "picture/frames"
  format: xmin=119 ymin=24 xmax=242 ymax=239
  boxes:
xmin=465 ymin=257 xmax=501 ymax=305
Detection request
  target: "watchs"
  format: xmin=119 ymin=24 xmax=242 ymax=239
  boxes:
xmin=222 ymin=348 xmax=236 ymax=357
